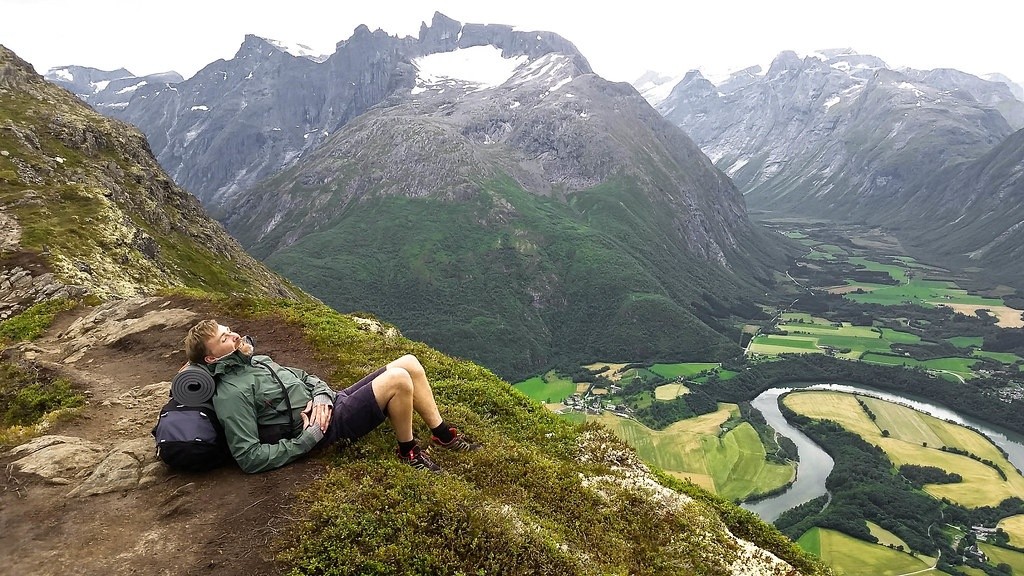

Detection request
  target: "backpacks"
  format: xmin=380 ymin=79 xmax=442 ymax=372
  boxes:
xmin=156 ymin=397 xmax=235 ymax=476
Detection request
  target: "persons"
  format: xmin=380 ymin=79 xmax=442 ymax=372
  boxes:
xmin=185 ymin=319 xmax=474 ymax=471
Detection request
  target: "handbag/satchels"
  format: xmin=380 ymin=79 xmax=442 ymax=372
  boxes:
xmin=256 ymin=421 xmax=296 ymax=445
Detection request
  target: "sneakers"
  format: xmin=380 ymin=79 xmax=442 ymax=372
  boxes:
xmin=431 ymin=427 xmax=483 ymax=452
xmin=396 ymin=445 xmax=449 ymax=476
